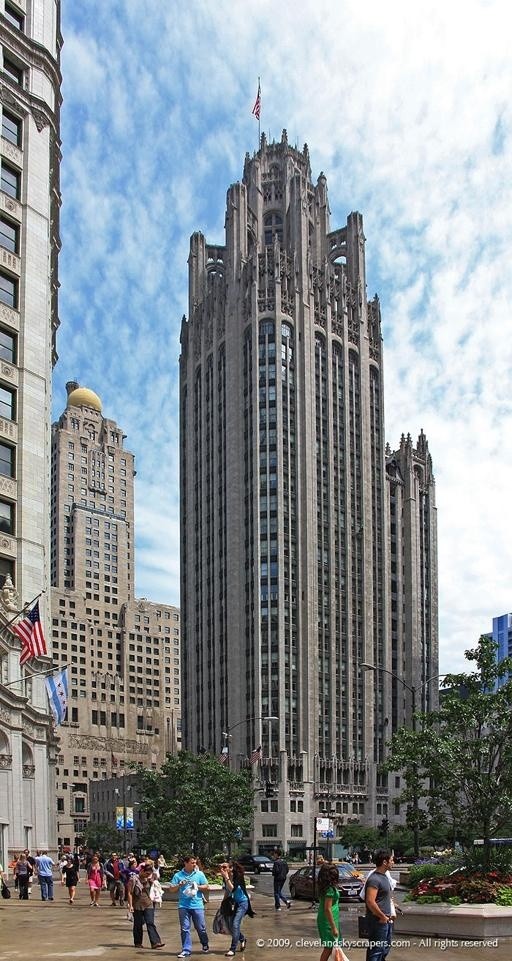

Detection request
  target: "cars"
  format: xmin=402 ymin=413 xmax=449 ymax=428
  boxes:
xmin=287 ymin=863 xmax=365 ymax=903
xmin=234 ymin=852 xmax=274 ymax=874
xmin=329 ymin=860 xmax=368 ymax=882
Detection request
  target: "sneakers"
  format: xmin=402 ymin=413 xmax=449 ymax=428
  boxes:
xmin=134 ymin=943 xmax=144 ymax=948
xmin=201 ymin=941 xmax=210 ymax=953
xmin=151 ymin=942 xmax=166 ymax=949
xmin=275 ymin=906 xmax=282 ymax=911
xmin=90 ymin=901 xmax=101 ymax=908
xmin=177 ymin=950 xmax=192 ymax=958
xmin=284 ymin=900 xmax=291 ymax=911
xmin=110 ymin=899 xmax=124 ymax=907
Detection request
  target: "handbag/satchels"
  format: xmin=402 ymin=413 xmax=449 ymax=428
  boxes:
xmin=220 ymin=896 xmax=237 ymax=916
xmin=212 ymin=909 xmax=233 ymax=936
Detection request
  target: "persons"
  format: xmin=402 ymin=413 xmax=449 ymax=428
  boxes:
xmin=2 ymin=842 xmax=403 ymax=960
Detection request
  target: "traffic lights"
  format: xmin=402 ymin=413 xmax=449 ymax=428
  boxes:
xmin=382 ymin=818 xmax=390 ymax=833
xmin=265 ymin=780 xmax=275 ymax=797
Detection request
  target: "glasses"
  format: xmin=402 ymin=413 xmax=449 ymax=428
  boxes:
xmin=221 ymin=866 xmax=227 ymax=869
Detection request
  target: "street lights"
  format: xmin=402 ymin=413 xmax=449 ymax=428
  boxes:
xmin=112 ymin=784 xmax=134 ymax=852
xmin=359 ymin=659 xmax=465 ymax=860
xmin=321 ymin=807 xmax=338 ymax=859
xmin=225 ymin=715 xmax=280 ymax=858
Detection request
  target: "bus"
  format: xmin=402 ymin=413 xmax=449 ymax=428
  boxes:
xmin=470 ymin=837 xmax=512 ymax=851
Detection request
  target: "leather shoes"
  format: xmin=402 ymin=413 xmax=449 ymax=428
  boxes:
xmin=224 ymin=950 xmax=236 ymax=957
xmin=240 ymin=938 xmax=247 ymax=951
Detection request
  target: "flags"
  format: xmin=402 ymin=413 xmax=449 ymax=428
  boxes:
xmin=12 ymin=598 xmax=47 ymax=668
xmin=44 ymin=670 xmax=69 ymax=727
